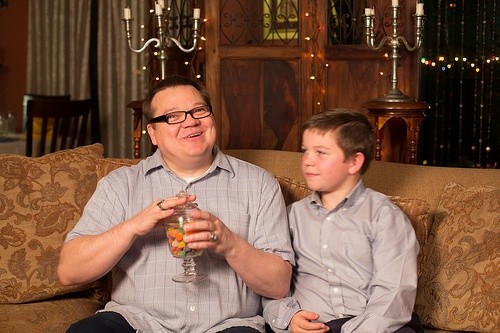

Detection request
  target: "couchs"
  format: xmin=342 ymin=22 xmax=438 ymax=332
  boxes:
xmin=0 ymin=149 xmax=500 ymax=333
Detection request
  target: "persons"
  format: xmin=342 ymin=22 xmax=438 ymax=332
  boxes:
xmin=56 ymin=76 xmax=297 ymax=333
xmin=262 ymin=108 xmax=425 ymax=333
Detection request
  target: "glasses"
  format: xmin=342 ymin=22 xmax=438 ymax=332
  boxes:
xmin=148 ymin=104 xmax=212 ymax=125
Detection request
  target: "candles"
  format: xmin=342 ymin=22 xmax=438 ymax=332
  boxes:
xmin=392 ymin=0 xmax=399 ymax=6
xmin=155 ymin=0 xmax=164 ymax=8
xmin=194 ymin=7 xmax=200 ymax=18
xmin=366 ymin=7 xmax=375 ymax=14
xmin=416 ymin=3 xmax=424 ymax=15
xmin=124 ymin=5 xmax=131 ymax=18
xmin=155 ymin=3 xmax=162 ymax=14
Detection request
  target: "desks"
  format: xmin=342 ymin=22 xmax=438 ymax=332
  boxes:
xmin=127 ymin=101 xmax=157 ymax=158
xmin=363 ymin=99 xmax=428 ymax=161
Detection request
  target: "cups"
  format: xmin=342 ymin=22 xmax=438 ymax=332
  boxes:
xmin=0 ymin=112 xmax=17 ymax=142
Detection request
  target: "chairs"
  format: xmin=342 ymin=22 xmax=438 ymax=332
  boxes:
xmin=25 ymin=94 xmax=95 ymax=157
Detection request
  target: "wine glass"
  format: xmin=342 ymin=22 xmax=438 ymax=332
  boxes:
xmin=163 ymin=191 xmax=209 ymax=283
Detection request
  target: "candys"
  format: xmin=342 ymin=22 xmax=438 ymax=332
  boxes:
xmin=166 ymin=228 xmax=203 ymax=257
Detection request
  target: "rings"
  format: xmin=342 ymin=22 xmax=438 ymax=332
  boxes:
xmin=210 ymin=232 xmax=217 ymax=241
xmin=157 ymin=200 xmax=164 ymax=210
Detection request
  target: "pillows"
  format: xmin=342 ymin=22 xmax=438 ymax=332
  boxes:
xmin=0 ymin=143 xmax=500 ymax=333
